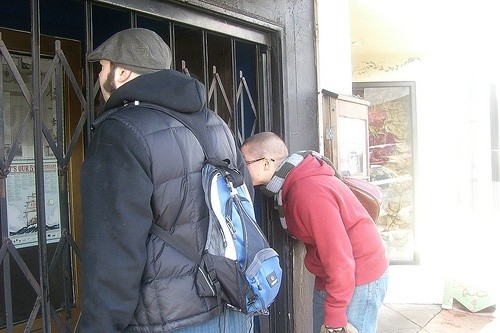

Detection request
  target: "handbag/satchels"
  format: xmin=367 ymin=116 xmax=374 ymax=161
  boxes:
xmin=340 ymin=174 xmax=385 ymax=225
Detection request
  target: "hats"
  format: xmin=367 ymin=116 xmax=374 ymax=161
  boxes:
xmin=88 ymin=28 xmax=172 ymax=74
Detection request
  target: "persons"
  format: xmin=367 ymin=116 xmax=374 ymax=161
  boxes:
xmin=71 ymin=28 xmax=257 ymax=333
xmin=241 ymin=131 xmax=390 ymax=332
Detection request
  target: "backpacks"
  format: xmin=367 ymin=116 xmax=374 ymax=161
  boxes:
xmin=88 ymin=101 xmax=283 ymax=316
